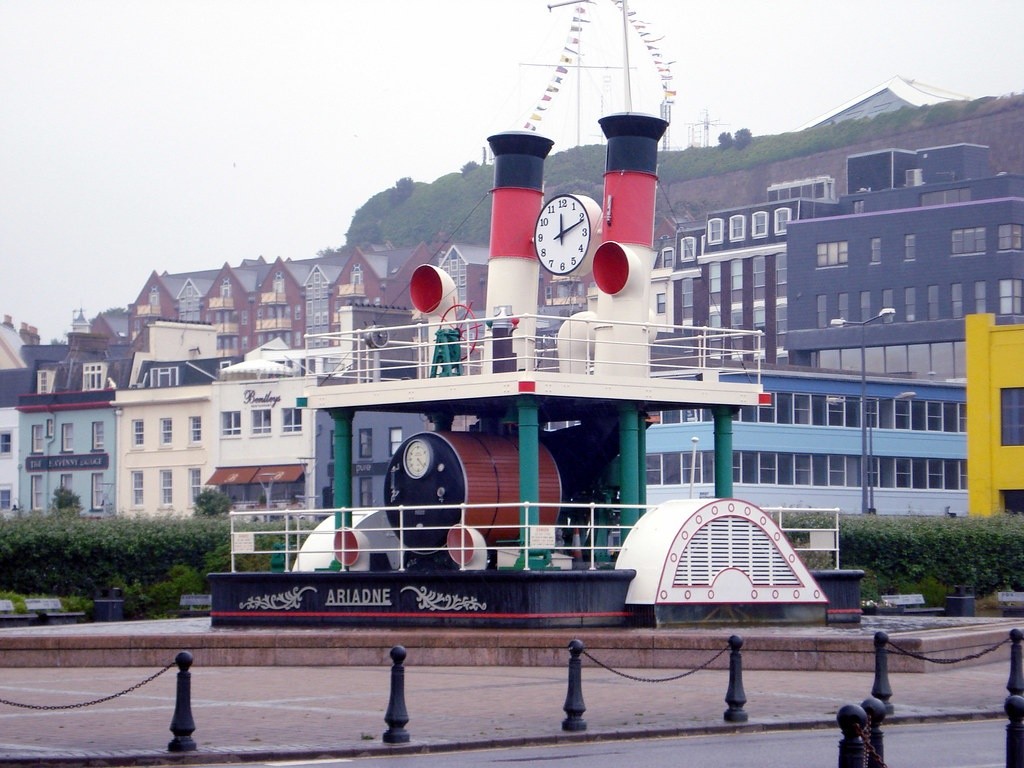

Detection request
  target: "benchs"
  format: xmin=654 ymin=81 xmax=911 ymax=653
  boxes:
xmin=0 ymin=600 xmax=38 ymax=627
xmin=881 ymin=595 xmax=944 ymax=616
xmin=24 ymin=598 xmax=85 ymax=624
xmin=168 ymin=594 xmax=211 ymax=617
xmin=997 ymin=592 xmax=1024 ymax=618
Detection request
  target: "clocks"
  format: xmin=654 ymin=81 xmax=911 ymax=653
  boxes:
xmin=533 ymin=193 xmax=603 ymax=275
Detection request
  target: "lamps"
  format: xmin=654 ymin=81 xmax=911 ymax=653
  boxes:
xmin=10 ymin=498 xmax=23 ymax=511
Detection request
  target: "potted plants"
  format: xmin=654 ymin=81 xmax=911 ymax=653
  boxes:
xmin=257 ymin=496 xmax=267 ymax=508
xmin=287 ymin=496 xmax=300 ymax=509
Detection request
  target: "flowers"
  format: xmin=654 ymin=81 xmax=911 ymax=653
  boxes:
xmin=861 ymin=600 xmax=877 ymax=608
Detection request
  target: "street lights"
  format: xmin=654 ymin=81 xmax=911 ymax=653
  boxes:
xmin=830 ymin=308 xmax=896 ymax=511
xmin=260 ymin=473 xmax=277 ymax=523
xmin=825 ymin=391 xmax=917 ymax=514
xmin=689 ymin=436 xmax=700 ymax=499
xmin=298 ymin=456 xmax=320 ymax=510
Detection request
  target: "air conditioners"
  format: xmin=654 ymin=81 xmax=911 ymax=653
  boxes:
xmin=906 ymin=169 xmax=922 ymax=186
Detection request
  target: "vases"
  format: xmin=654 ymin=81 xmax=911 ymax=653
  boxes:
xmin=862 ymin=608 xmax=876 ymax=616
